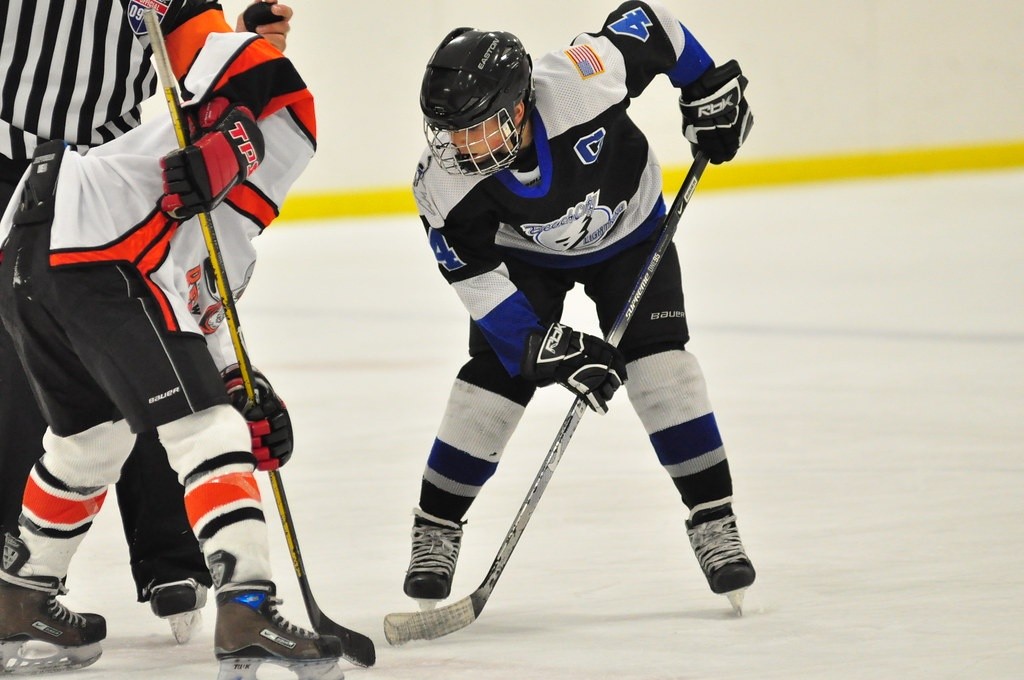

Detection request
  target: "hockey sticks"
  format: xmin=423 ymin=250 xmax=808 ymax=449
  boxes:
xmin=137 ymin=1 xmax=376 ymax=668
xmin=384 ymin=143 xmax=712 ymax=647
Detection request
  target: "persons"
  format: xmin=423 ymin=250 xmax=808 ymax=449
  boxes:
xmin=0 ymin=1 xmax=344 ymax=664
xmin=401 ymin=0 xmax=754 ymax=599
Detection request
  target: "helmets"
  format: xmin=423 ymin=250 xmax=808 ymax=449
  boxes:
xmin=419 ymin=28 xmax=527 ymax=172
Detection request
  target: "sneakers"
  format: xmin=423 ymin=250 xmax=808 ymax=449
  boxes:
xmin=403 ymin=508 xmax=468 ymax=611
xmin=147 ymin=577 xmax=208 ymax=644
xmin=214 ymin=580 xmax=346 ymax=680
xmin=685 ymin=501 xmax=755 ymax=617
xmin=0 ymin=532 xmax=107 ymax=676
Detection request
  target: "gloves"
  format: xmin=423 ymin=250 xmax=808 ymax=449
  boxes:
xmin=225 ymin=369 xmax=293 ymax=472
xmin=524 ymin=321 xmax=628 ymax=416
xmin=159 ymin=108 xmax=266 ymax=220
xmin=680 ymin=60 xmax=754 ymax=164
